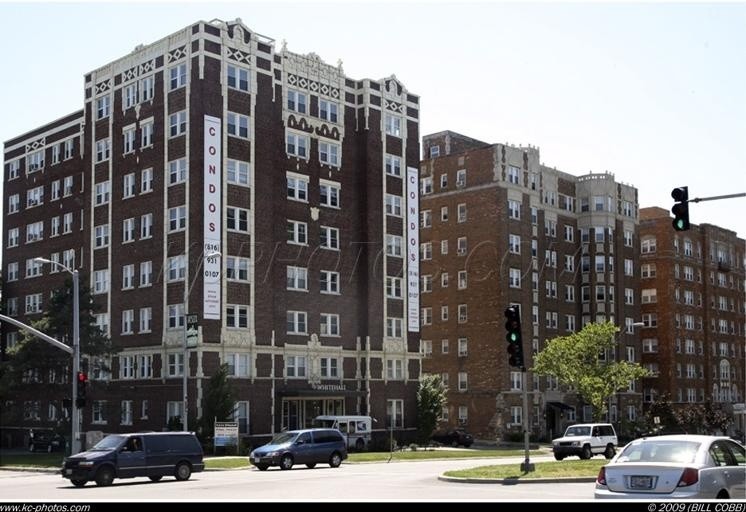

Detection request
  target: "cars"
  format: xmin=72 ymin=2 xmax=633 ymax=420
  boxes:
xmin=445 ymin=429 xmax=473 ymax=447
xmin=594 ymin=433 xmax=746 ymax=500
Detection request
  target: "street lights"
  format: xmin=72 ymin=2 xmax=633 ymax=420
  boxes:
xmin=183 ymin=253 xmax=222 ymax=432
xmin=608 ymin=322 xmax=646 ymax=422
xmin=34 ymin=257 xmax=81 ymax=455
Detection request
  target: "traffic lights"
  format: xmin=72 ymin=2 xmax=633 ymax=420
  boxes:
xmin=671 ymin=186 xmax=690 ymax=232
xmin=77 ymin=372 xmax=88 ymax=397
xmin=504 ymin=307 xmax=522 ymax=366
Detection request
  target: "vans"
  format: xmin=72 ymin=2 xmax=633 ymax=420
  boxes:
xmin=311 ymin=414 xmax=373 ymax=451
xmin=251 ymin=427 xmax=348 ymax=470
xmin=62 ymin=431 xmax=206 ymax=487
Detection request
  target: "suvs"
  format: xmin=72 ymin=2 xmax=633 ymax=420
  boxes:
xmin=551 ymin=422 xmax=618 ymax=458
